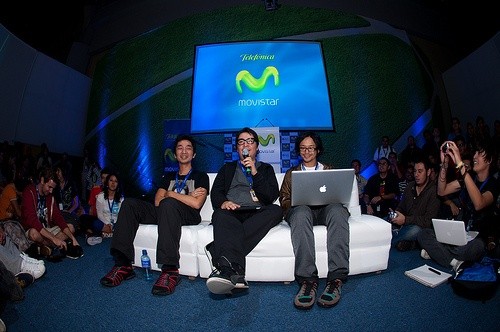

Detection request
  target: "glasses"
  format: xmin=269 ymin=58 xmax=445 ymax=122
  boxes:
xmin=298 ymin=145 xmax=318 ymax=151
xmin=237 ymin=138 xmax=256 ymax=145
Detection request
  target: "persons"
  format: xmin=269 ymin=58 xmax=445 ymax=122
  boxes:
xmin=100 ymin=135 xmax=208 ymax=296
xmin=0 ymin=139 xmax=124 ymax=332
xmin=205 ymin=128 xmax=282 ymax=295
xmin=350 ymin=114 xmax=500 ymax=289
xmin=278 ymin=131 xmax=350 ymax=310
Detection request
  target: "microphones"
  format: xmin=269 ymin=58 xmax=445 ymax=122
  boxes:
xmin=243 ymin=148 xmax=251 ymax=174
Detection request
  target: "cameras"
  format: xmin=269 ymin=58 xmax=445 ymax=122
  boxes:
xmin=389 ymin=212 xmax=397 ymax=219
xmin=442 ymin=142 xmax=452 ymax=153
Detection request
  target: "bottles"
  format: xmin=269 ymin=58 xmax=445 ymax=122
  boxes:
xmin=141 ymin=250 xmax=153 ymax=281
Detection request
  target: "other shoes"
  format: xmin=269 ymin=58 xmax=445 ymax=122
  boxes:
xmin=421 ymin=249 xmax=431 ymax=259
xmin=454 ymin=261 xmax=464 ymax=272
xmin=87 ymin=236 xmax=102 ymax=245
xmin=396 ymin=240 xmax=411 ymax=252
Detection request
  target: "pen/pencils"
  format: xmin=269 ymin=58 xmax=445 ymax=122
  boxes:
xmin=428 ymin=268 xmax=442 ymax=275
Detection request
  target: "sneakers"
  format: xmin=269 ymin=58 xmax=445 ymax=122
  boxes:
xmin=151 ymin=267 xmax=181 ymax=295
xmin=100 ymin=264 xmax=136 ymax=287
xmin=234 ymin=275 xmax=249 ymax=288
xmin=317 ymin=279 xmax=342 ymax=308
xmin=15 ymin=252 xmax=46 ymax=279
xmin=43 ymin=246 xmax=53 ymax=256
xmin=294 ymin=281 xmax=317 ymax=310
xmin=14 ymin=272 xmax=34 ymax=288
xmin=206 ymin=272 xmax=236 ymax=294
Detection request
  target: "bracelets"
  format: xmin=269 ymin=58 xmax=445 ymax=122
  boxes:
xmin=461 ymin=164 xmax=469 ymax=179
xmin=455 ymin=160 xmax=464 ymax=172
xmin=439 ymin=164 xmax=448 ymax=169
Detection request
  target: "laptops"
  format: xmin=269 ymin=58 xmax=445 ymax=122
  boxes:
xmin=432 ymin=219 xmax=479 ymax=246
xmin=291 ymin=169 xmax=355 ymax=206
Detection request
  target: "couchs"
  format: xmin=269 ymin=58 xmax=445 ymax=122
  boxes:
xmin=132 ymin=172 xmax=392 ymax=284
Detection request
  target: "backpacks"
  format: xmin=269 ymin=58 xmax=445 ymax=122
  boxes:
xmin=448 ymin=256 xmax=500 ymax=304
